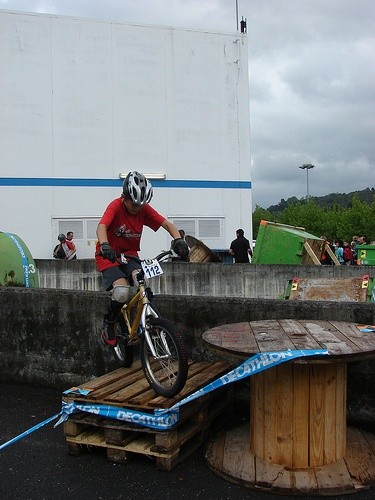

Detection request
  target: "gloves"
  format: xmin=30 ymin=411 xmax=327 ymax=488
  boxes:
xmin=173 ymin=237 xmax=189 ymax=257
xmin=100 ymin=243 xmax=117 ymax=264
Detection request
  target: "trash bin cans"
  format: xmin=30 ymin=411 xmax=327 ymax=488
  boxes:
xmin=0 ymin=231 xmax=40 ymax=288
xmin=355 ymin=245 xmax=375 ymax=300
xmin=252 ymin=220 xmax=327 ymax=299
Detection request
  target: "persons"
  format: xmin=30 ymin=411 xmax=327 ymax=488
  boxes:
xmin=320 ymin=235 xmax=367 ymax=265
xmin=229 ymin=229 xmax=253 ymax=263
xmin=53 ymin=232 xmax=77 ymax=260
xmin=168 ymin=229 xmax=190 ymax=263
xmin=95 ymin=172 xmax=190 ymax=351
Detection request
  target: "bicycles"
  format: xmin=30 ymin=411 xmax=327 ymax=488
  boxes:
xmin=98 ymin=249 xmax=189 ymax=399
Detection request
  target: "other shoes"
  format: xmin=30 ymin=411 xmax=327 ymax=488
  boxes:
xmin=102 ymin=313 xmax=118 ymax=347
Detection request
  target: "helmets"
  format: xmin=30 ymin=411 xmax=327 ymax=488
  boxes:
xmin=58 ymin=233 xmax=66 ymax=241
xmin=124 ymin=171 xmax=153 ymax=205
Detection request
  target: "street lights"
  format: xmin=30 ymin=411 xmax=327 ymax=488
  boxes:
xmin=298 ymin=163 xmax=315 ymax=203
